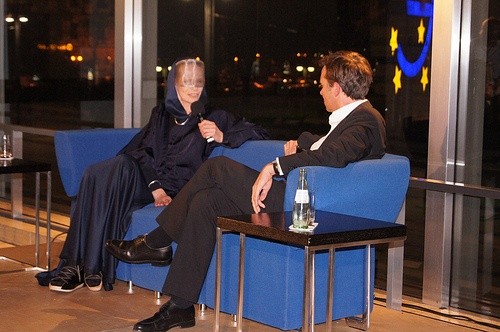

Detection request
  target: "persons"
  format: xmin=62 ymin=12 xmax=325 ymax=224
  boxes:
xmin=48 ymin=57 xmax=273 ymax=292
xmin=103 ymin=49 xmax=386 ymax=332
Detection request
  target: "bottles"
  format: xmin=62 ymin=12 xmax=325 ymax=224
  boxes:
xmin=292 ymin=168 xmax=309 ymax=229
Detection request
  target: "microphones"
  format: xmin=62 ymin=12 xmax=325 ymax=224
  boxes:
xmin=190 ymin=100 xmax=215 ymax=143
xmin=295 ymin=132 xmax=315 ymax=154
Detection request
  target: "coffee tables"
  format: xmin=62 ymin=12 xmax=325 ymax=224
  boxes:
xmin=215 ymin=209 xmax=407 ymax=332
xmin=0 ymin=158 xmax=51 ymax=270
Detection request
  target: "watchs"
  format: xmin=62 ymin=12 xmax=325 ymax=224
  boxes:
xmin=272 ymin=159 xmax=280 ymax=176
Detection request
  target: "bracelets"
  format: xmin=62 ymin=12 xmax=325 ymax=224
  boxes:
xmin=148 ymin=180 xmax=161 ymax=188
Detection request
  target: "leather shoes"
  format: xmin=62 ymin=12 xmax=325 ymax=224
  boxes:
xmin=132 ymin=300 xmax=196 ymax=332
xmin=104 ymin=232 xmax=173 ymax=267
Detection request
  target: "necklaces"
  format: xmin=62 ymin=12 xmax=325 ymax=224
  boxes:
xmin=174 ymin=113 xmax=192 ymax=125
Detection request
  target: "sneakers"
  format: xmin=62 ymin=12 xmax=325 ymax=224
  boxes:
xmin=48 ymin=265 xmax=85 ymax=293
xmin=83 ymin=269 xmax=103 ymax=292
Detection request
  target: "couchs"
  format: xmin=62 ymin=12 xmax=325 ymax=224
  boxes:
xmin=55 ymin=128 xmax=411 ymax=332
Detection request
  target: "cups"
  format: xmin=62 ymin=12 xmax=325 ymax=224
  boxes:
xmin=309 ymin=192 xmax=316 ymax=226
xmin=0 ymin=135 xmax=13 ymax=160
